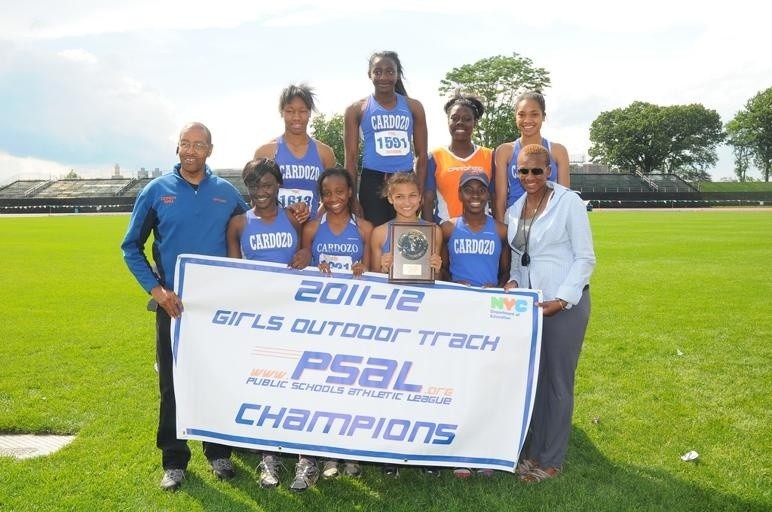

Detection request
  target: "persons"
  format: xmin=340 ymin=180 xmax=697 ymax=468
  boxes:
xmin=121 ymin=122 xmax=596 ymax=488
xmin=250 ymin=50 xmax=570 ymax=225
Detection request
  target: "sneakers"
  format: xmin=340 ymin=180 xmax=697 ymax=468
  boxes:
xmin=206 ymin=457 xmax=236 ymax=482
xmin=342 ymin=461 xmax=362 ymax=479
xmin=259 ymin=451 xmax=282 ymax=488
xmin=453 ymin=467 xmax=472 ymax=477
xmin=321 ymin=459 xmax=340 ymax=480
xmin=159 ymin=468 xmax=185 ymax=492
xmin=476 ymin=468 xmax=494 ymax=477
xmin=382 ymin=463 xmax=400 ymax=480
xmin=290 ymin=457 xmax=322 ymax=490
xmin=426 ymin=466 xmax=440 ymax=478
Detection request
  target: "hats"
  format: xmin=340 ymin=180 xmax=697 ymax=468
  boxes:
xmin=459 ymin=167 xmax=490 ymax=188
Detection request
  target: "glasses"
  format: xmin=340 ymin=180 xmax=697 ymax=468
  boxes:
xmin=516 ymin=164 xmax=548 ymax=176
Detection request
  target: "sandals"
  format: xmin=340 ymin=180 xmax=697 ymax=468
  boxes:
xmin=511 ymin=458 xmax=539 ymax=476
xmin=520 ymin=466 xmax=559 ymax=484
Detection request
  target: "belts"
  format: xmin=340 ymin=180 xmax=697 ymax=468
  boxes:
xmin=583 ymin=283 xmax=589 ymax=292
